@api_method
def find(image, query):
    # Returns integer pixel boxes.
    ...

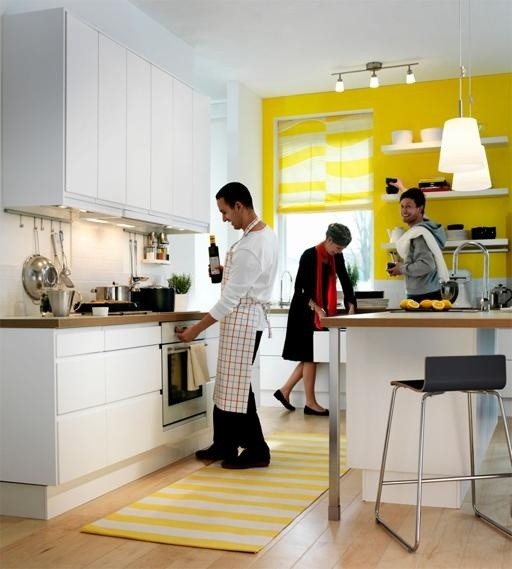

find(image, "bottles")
[495,283,512,308]
[144,231,170,260]
[208,235,222,283]
[131,287,140,303]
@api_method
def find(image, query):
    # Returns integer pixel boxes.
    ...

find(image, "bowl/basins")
[386,178,399,194]
[392,130,412,144]
[472,227,496,239]
[420,128,442,142]
[446,224,466,240]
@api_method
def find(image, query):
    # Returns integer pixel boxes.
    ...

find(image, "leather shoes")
[274,389,295,410]
[304,405,329,415]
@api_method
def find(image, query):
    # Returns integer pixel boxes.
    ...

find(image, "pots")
[22,227,58,305]
[91,281,134,302]
[140,280,176,313]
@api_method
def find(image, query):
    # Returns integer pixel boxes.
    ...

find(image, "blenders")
[440,269,472,308]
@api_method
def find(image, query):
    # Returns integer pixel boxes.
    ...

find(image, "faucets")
[453,241,490,312]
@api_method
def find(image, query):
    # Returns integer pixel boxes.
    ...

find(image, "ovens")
[161,319,208,433]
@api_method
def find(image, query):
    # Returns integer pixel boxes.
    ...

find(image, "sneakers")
[222,450,270,469]
[195,443,238,460]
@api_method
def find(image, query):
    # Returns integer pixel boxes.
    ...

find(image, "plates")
[354,291,389,310]
[418,177,451,192]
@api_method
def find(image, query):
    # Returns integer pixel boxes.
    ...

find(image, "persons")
[273,223,356,415]
[385,178,447,303]
[178,182,279,470]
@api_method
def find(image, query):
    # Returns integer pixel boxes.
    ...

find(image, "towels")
[185,343,209,391]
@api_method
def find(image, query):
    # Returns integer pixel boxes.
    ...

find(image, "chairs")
[372,355,511,553]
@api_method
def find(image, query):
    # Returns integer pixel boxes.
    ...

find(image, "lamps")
[452,1,491,193]
[437,1,482,174]
[330,61,420,92]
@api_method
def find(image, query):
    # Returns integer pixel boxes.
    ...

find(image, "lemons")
[399,299,406,309]
[431,299,445,311]
[406,299,420,309]
[420,299,433,309]
[440,299,452,310]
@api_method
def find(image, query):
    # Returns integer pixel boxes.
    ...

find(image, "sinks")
[391,308,480,313]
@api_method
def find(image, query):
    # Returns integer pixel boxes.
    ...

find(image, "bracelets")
[311,303,316,311]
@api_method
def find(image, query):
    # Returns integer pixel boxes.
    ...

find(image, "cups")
[39,288,83,318]
[92,306,109,316]
[14,301,27,316]
[478,123,488,138]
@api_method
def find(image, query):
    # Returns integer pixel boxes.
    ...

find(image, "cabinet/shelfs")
[207,321,260,425]
[53,323,164,487]
[0,6,126,222]
[98,45,172,233]
[172,73,211,233]
[380,136,509,254]
[260,311,346,409]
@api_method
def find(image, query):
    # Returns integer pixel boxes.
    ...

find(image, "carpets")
[78,430,350,555]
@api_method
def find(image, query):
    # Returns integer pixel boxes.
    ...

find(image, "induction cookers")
[72,302,139,314]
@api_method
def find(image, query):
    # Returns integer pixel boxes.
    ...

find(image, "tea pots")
[386,225,406,244]
